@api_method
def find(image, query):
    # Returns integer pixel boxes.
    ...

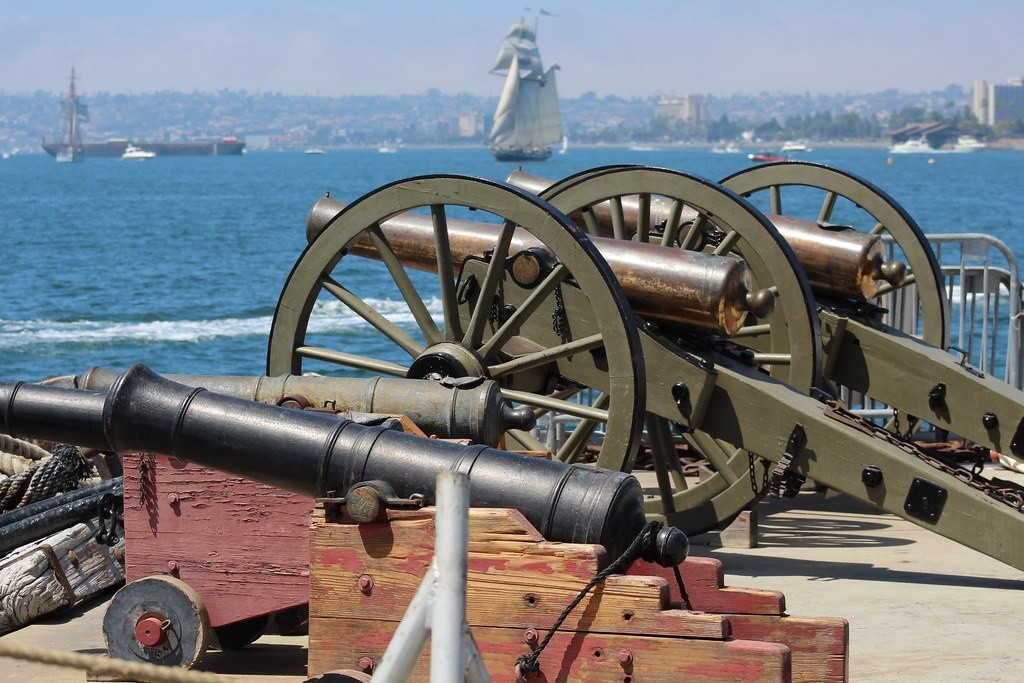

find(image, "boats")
[892,140,932,154]
[954,135,984,148]
[123,145,155,159]
[301,142,814,157]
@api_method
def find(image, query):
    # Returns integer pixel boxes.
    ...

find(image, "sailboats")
[42,65,244,157]
[489,10,565,161]
